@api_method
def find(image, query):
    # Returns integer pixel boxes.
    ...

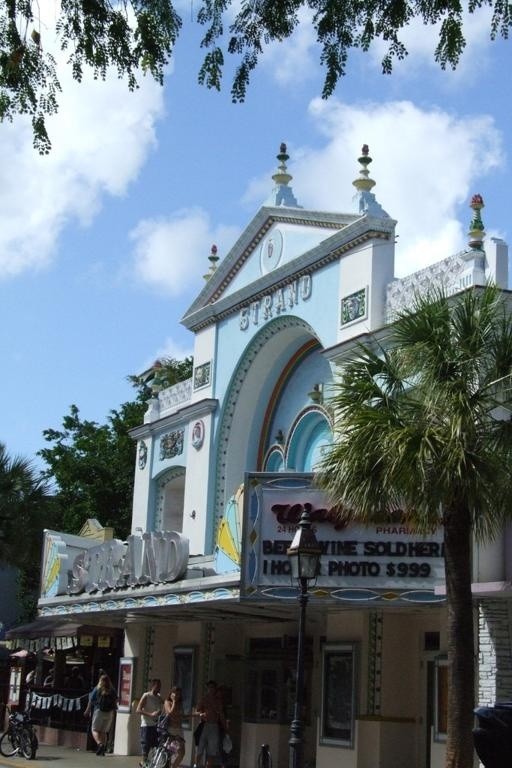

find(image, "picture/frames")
[338,284,368,330]
[169,644,197,731]
[318,639,359,750]
[193,358,211,393]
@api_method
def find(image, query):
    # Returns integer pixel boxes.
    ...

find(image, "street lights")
[286,507,321,768]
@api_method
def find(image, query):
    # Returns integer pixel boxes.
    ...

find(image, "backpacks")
[93,687,115,713]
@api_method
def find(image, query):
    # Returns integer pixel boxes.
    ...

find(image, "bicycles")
[0,703,39,760]
[143,726,186,768]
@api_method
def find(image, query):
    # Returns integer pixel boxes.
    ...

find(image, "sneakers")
[96,743,105,756]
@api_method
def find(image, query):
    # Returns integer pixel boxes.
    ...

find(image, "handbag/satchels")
[156,714,169,732]
[222,734,232,754]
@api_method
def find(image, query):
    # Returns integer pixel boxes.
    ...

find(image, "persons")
[134,676,166,768]
[161,686,199,767]
[196,678,232,767]
[23,661,118,756]
